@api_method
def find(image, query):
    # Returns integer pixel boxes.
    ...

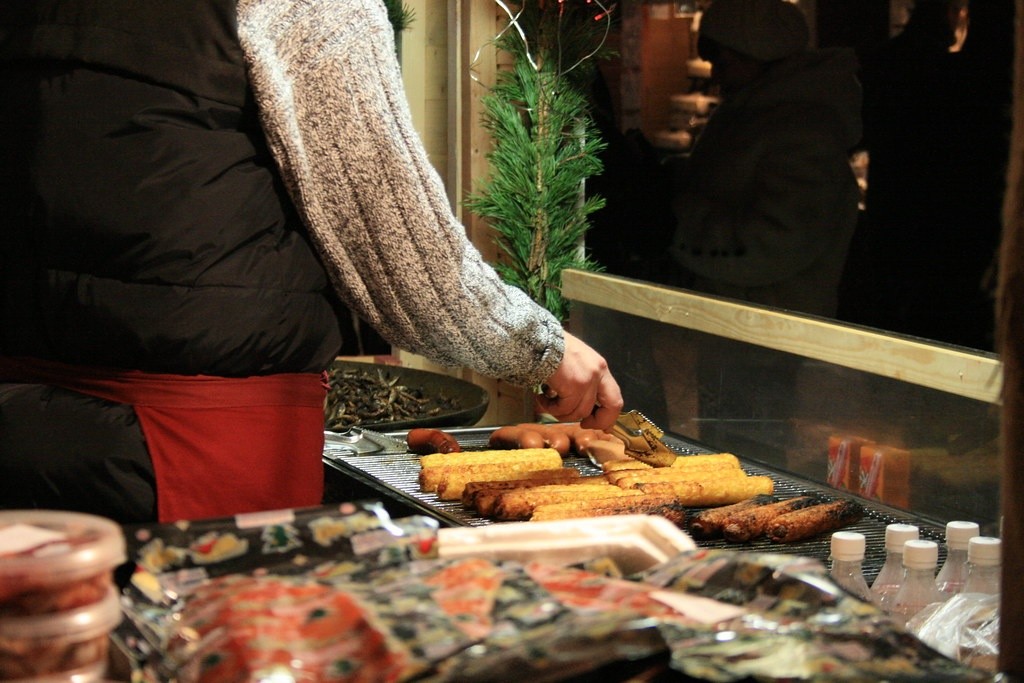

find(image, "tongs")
[542,384,675,468]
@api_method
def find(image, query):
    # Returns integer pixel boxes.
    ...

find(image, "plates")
[323,360,487,433]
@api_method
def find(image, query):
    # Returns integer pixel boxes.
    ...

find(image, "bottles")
[830,531,871,602]
[869,524,919,613]
[935,521,980,596]
[890,540,941,626]
[962,536,1002,618]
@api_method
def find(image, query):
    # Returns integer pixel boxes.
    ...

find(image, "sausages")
[404,418,627,456]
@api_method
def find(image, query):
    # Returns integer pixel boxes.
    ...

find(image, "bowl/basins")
[0,511,123,683]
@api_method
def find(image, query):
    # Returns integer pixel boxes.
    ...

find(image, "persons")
[676,1,865,464]
[0,0,624,527]
